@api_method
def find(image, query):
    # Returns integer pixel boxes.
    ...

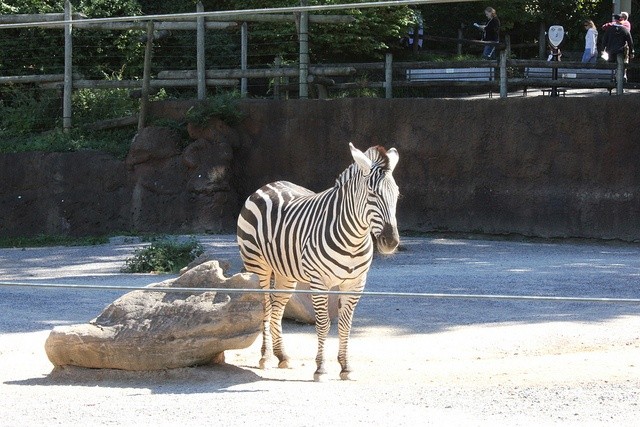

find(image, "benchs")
[406,67,494,98]
[522,67,627,96]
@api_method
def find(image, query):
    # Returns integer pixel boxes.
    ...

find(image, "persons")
[478,6,501,60]
[601,11,632,63]
[599,14,636,96]
[399,4,424,51]
[547,49,563,61]
[581,18,599,81]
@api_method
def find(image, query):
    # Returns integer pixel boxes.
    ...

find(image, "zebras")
[236,141,403,382]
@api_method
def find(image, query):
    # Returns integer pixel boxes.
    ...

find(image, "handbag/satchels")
[495,43,506,50]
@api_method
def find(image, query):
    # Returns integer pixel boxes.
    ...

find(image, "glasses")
[584,25,587,26]
